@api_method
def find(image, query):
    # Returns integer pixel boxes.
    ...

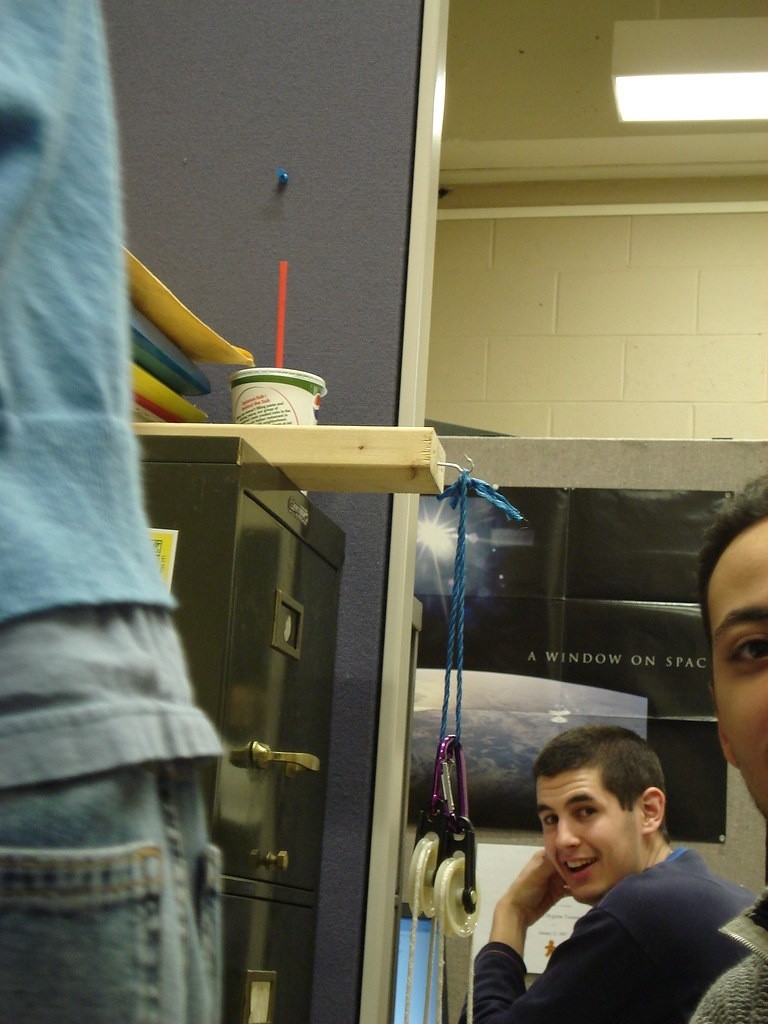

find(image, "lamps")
[611,0,768,123]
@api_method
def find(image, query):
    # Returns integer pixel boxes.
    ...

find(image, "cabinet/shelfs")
[134,435,346,1024]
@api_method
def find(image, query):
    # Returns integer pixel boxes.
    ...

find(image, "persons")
[687,469,768,1024]
[0,0,231,1024]
[456,725,756,1024]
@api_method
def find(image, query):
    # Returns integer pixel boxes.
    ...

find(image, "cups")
[229,368,328,425]
